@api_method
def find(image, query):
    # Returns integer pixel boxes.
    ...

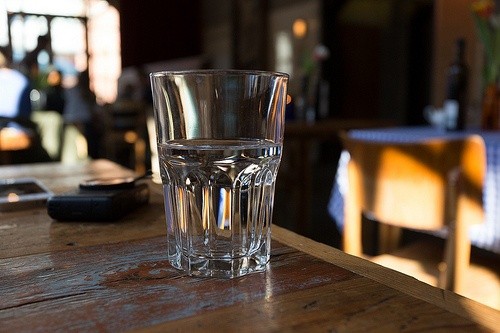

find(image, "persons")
[0,34,143,165]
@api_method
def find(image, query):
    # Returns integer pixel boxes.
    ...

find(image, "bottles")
[445,38,468,132]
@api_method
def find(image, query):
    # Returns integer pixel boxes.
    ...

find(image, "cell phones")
[0,178,53,212]
[47,183,149,222]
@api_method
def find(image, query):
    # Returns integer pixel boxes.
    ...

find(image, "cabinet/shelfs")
[110,1,434,241]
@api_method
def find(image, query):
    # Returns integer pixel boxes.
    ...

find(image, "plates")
[0,182,51,208]
[81,178,136,189]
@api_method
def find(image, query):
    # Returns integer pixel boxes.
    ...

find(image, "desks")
[0,164,500,333]
[342,129,500,294]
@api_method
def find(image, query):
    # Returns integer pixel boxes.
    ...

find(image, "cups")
[149,69,289,279]
